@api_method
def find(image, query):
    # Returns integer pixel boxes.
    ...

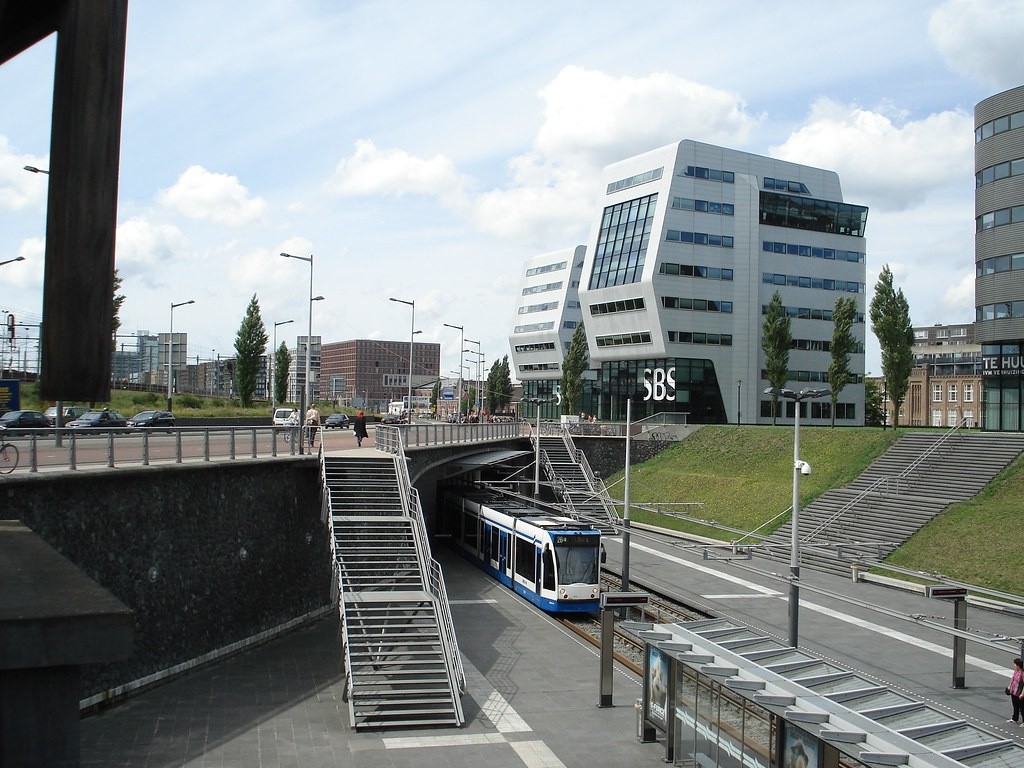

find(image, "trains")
[439,483,607,613]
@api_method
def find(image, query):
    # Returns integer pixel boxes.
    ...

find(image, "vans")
[44,406,91,427]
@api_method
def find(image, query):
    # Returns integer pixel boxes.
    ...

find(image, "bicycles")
[0,426,19,474]
[282,419,298,443]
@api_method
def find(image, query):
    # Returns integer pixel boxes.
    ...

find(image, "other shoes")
[358,442,361,447]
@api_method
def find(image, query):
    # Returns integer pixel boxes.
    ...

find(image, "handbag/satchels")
[312,421,318,428]
[353,431,357,436]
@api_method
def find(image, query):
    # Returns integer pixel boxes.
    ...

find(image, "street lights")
[389,298,423,432]
[449,338,489,415]
[735,380,745,427]
[168,300,196,412]
[761,386,832,648]
[272,320,295,418]
[280,252,325,438]
[519,397,558,508]
[442,324,464,423]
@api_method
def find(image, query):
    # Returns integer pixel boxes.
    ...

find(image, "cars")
[124,410,176,435]
[0,410,52,438]
[65,412,127,436]
[380,414,398,424]
[447,413,458,423]
[273,409,299,436]
[325,413,350,430]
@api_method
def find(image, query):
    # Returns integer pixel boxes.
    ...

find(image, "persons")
[1004,657,1024,727]
[305,403,321,448]
[287,408,300,427]
[353,410,368,447]
